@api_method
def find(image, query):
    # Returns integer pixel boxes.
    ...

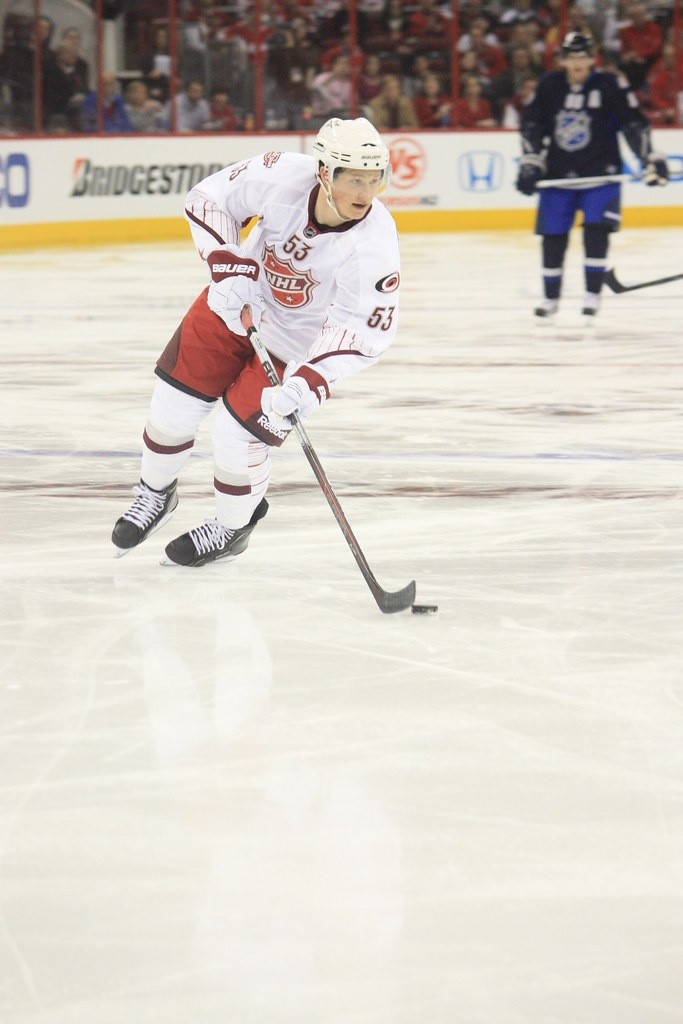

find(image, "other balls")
[411,604,439,615]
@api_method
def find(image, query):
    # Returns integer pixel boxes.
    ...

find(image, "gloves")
[207,247,264,336]
[515,163,542,196]
[261,359,332,431]
[644,157,669,186]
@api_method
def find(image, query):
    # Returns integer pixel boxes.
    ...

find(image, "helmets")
[311,116,390,195]
[558,28,597,59]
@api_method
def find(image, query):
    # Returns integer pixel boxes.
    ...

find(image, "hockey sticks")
[240,307,417,615]
[532,169,683,190]
[606,266,683,295]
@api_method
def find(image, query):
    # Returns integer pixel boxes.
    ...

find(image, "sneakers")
[107,478,181,557]
[159,500,270,573]
[534,294,560,317]
[580,292,603,315]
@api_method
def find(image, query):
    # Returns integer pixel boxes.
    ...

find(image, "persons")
[157,0,682,130]
[161,81,210,133]
[123,80,171,134]
[81,73,130,132]
[518,32,671,323]
[0,15,54,132]
[41,26,90,133]
[146,24,181,103]
[203,91,236,130]
[113,117,401,565]
